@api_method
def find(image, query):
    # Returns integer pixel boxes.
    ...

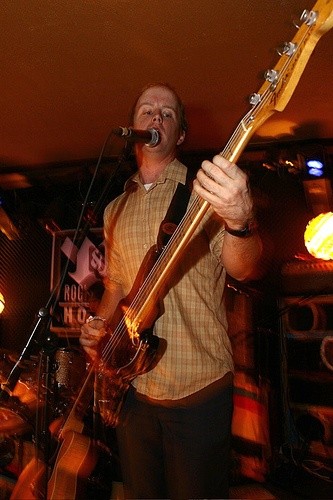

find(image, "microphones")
[113,125,159,147]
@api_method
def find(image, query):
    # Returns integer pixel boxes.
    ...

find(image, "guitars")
[9,365,93,500]
[89,0,333,429]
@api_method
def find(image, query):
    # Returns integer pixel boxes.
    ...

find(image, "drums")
[47,346,88,391]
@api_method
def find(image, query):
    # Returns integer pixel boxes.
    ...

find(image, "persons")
[78,83,276,500]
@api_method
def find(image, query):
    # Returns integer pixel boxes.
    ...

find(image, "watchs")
[225,218,258,238]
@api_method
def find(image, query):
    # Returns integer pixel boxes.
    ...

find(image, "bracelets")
[86,316,107,324]
